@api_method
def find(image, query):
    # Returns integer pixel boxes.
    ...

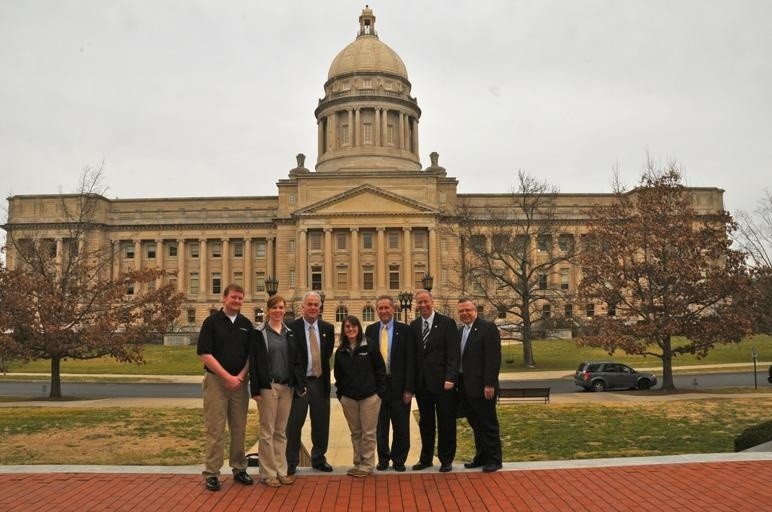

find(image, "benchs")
[496,388,550,403]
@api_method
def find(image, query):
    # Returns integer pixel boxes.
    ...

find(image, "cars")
[768,365,772,384]
[574,361,656,391]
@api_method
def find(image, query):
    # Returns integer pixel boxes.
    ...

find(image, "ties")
[380,325,388,365]
[422,321,430,349]
[309,326,321,378]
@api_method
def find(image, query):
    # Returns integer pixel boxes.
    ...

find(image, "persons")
[333,316,386,477]
[365,295,416,472]
[197,284,253,490]
[457,296,503,472]
[249,295,307,487]
[410,290,458,472]
[287,292,333,475]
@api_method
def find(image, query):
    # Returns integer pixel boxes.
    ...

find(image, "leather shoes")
[206,461,502,490]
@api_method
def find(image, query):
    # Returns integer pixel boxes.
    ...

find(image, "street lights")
[399,291,412,324]
